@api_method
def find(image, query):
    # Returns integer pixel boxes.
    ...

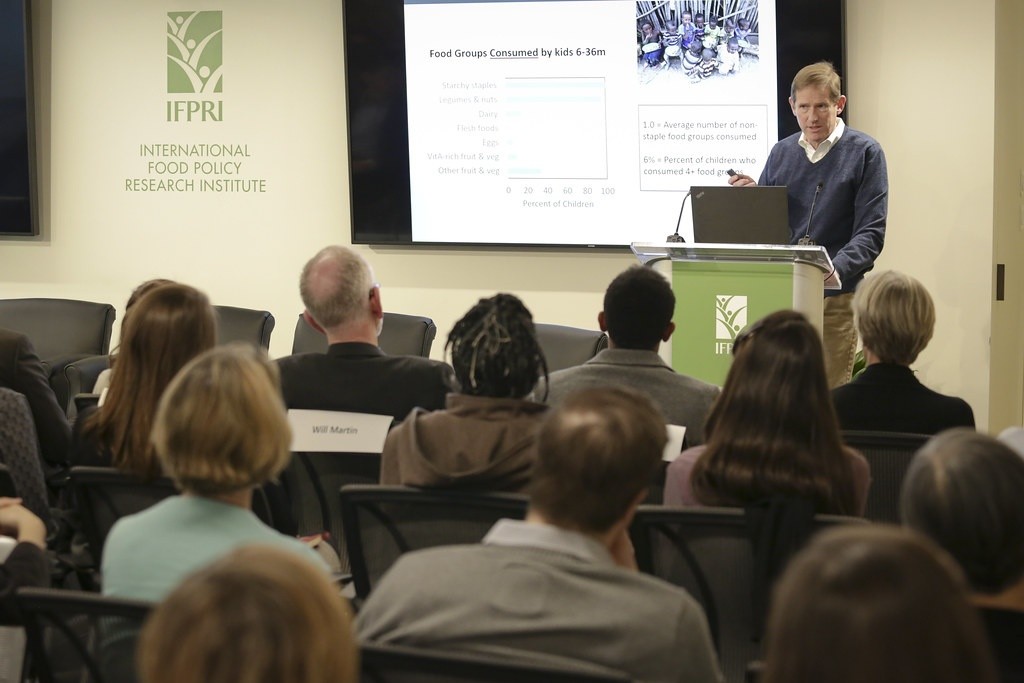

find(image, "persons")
[73,284,218,471]
[663,311,872,517]
[899,426,1024,683]
[832,270,976,434]
[0,329,70,636]
[357,385,725,683]
[639,11,751,79]
[728,64,889,391]
[274,247,452,428]
[100,342,343,602]
[380,293,554,492]
[533,266,718,449]
[135,536,357,682]
[763,523,1002,683]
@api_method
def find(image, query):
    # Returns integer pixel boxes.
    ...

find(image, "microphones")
[666,191,692,244]
[799,183,824,244]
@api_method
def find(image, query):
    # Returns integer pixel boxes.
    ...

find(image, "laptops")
[691,185,790,246]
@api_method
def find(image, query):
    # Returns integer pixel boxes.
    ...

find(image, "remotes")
[727,169,739,181]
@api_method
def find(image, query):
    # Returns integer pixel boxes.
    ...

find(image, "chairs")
[0,297,1024,683]
